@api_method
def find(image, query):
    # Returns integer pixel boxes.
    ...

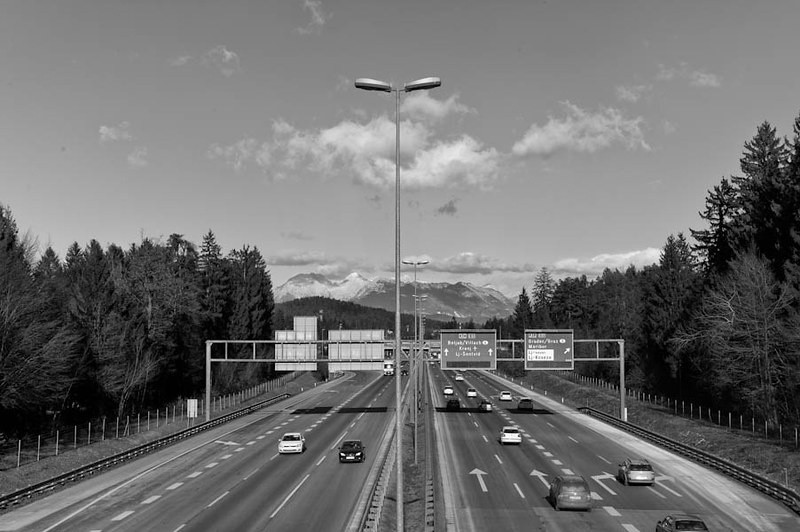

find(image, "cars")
[499,391,513,402]
[500,426,522,445]
[477,400,492,412]
[548,474,592,512]
[455,373,464,381]
[278,432,306,454]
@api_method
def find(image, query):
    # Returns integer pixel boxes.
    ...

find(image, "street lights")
[356,75,442,526]
[401,260,429,465]
[412,294,428,411]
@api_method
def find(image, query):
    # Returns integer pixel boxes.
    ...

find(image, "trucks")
[383,360,395,376]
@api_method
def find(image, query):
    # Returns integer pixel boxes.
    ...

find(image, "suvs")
[446,399,460,412]
[517,397,533,411]
[443,386,454,395]
[465,388,478,398]
[337,440,366,463]
[616,456,655,487]
[655,513,710,532]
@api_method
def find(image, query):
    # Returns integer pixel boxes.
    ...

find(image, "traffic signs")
[524,329,574,371]
[440,329,497,371]
